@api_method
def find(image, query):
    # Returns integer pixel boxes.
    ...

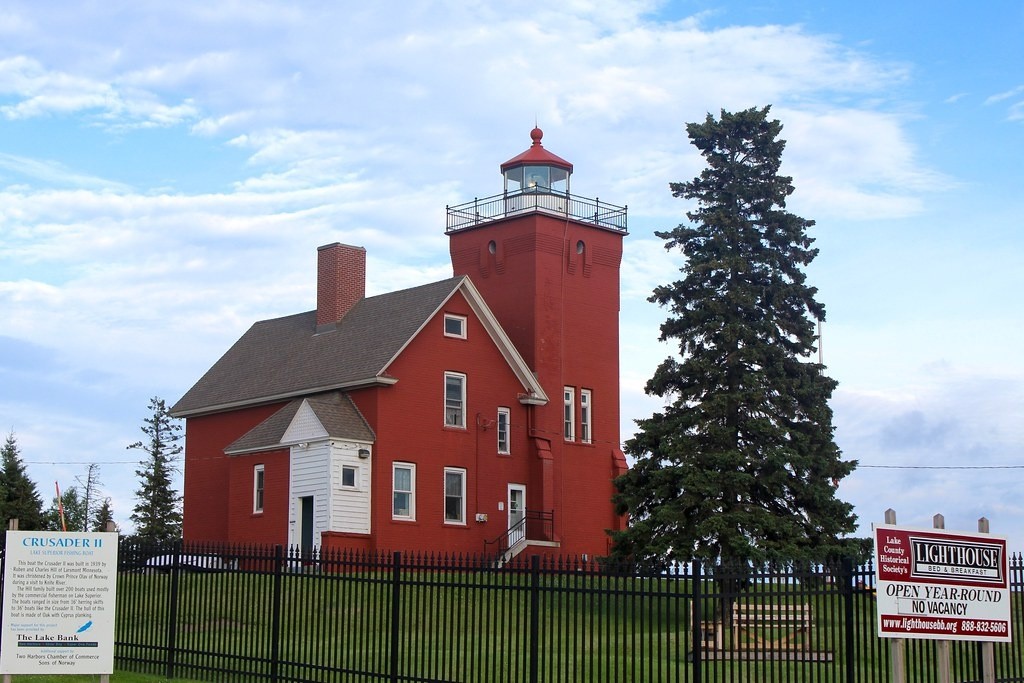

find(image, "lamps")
[359,449,371,458]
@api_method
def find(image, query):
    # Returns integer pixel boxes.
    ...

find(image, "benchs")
[733,601,815,651]
[690,600,723,651]
